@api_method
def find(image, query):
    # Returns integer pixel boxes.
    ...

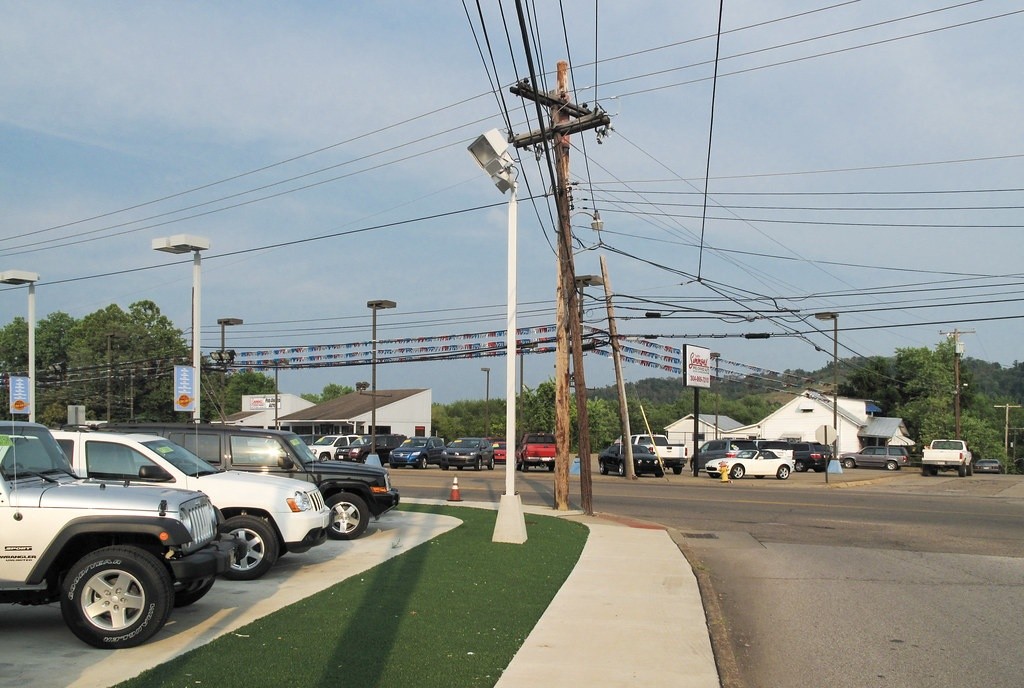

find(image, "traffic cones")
[444,471,465,502]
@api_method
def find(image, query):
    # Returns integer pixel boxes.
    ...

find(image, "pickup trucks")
[515,432,556,472]
[921,439,973,477]
[614,434,690,475]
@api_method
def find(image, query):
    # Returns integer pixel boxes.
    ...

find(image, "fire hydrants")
[717,461,732,484]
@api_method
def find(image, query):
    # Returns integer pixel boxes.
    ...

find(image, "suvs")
[839,446,911,470]
[790,441,835,472]
[46,422,335,581]
[690,439,758,471]
[0,419,249,649]
[752,438,796,466]
[292,434,506,472]
[98,417,400,541]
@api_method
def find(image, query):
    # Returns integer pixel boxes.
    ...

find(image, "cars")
[704,449,794,479]
[973,458,1006,475]
[598,443,666,477]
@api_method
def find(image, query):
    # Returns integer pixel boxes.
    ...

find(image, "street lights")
[575,274,605,344]
[518,343,539,440]
[151,234,212,424]
[710,352,721,440]
[272,359,290,431]
[480,366,491,439]
[466,127,530,543]
[814,312,839,459]
[0,268,40,424]
[105,332,126,424]
[366,299,398,454]
[215,318,244,423]
